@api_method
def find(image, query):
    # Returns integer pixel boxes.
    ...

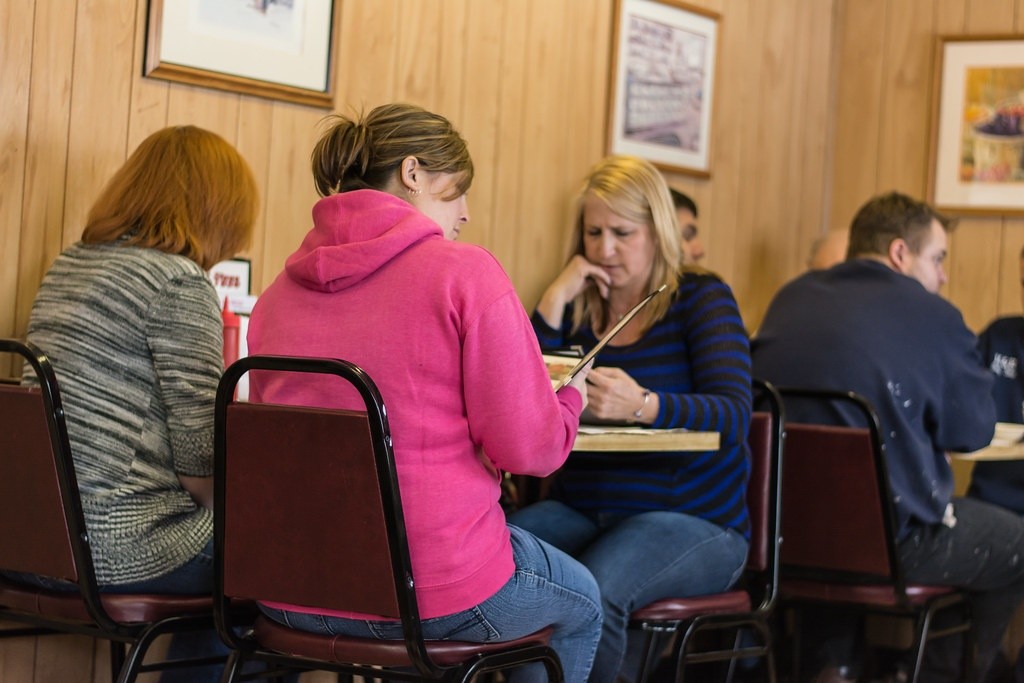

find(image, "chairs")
[624,377,786,683]
[0,336,302,683]
[775,387,978,683]
[211,354,565,683]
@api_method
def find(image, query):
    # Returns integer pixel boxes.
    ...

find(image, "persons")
[967,316,1024,514]
[751,192,1024,682]
[249,103,603,682]
[668,187,705,266]
[17,126,302,683]
[501,154,752,683]
[808,226,850,271]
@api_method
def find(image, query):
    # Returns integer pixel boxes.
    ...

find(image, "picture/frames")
[602,0,724,181]
[142,0,343,111]
[923,33,1024,221]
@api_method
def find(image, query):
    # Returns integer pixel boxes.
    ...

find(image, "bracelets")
[626,388,650,426]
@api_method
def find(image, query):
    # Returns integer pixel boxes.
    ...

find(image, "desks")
[944,443,1024,501]
[498,424,719,522]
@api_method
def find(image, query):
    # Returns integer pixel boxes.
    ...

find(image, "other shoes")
[811,659,860,683]
